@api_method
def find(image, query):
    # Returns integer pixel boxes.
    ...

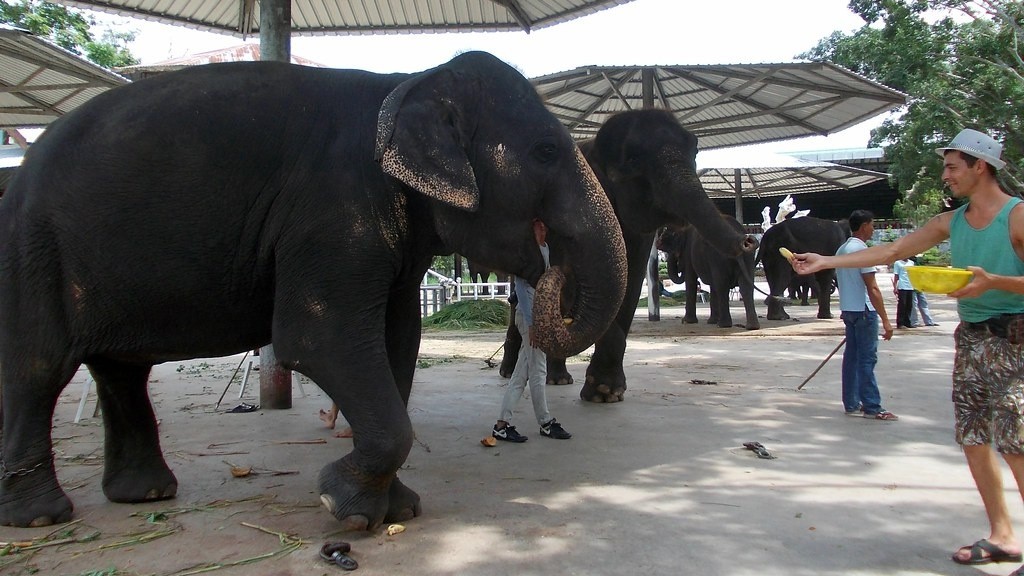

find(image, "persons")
[317,398,354,439]
[893,255,915,329]
[785,127,1024,576]
[492,218,572,442]
[908,256,939,328]
[831,209,899,420]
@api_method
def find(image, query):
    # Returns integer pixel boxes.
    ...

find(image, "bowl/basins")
[904,266,973,293]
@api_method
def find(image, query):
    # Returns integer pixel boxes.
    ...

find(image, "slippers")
[226,402,259,413]
[844,405,865,415]
[952,538,1023,565]
[863,411,898,420]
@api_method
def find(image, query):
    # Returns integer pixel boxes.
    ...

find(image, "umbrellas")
[520,58,911,321]
[693,154,894,229]
[1,23,133,132]
[43,1,637,412]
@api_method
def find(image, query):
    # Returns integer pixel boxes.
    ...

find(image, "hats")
[934,129,1007,171]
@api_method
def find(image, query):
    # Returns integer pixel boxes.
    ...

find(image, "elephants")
[0,50,854,533]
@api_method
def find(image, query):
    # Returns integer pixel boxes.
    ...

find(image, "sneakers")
[540,417,572,440]
[492,422,528,443]
